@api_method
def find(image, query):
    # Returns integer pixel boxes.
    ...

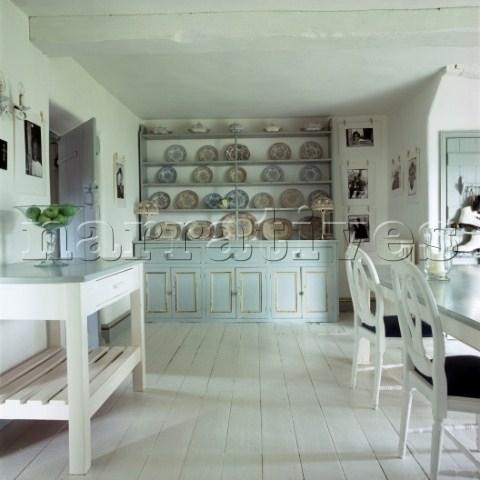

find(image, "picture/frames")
[345,212,373,244]
[390,162,402,192]
[343,125,377,150]
[25,120,43,178]
[405,154,419,195]
[113,152,126,200]
[342,165,375,206]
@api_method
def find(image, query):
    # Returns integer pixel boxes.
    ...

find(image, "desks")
[358,264,480,365]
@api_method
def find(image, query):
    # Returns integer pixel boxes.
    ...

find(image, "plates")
[150,143,258,240]
[259,141,335,240]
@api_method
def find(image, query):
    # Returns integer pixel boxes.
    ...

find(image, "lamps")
[135,200,159,240]
[12,82,31,120]
[312,196,335,240]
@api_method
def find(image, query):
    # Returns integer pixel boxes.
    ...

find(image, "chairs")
[344,245,433,410]
[390,258,480,480]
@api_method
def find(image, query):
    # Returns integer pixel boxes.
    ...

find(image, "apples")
[26,203,77,228]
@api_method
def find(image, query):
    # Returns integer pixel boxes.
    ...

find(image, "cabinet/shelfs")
[132,119,340,324]
[0,256,146,474]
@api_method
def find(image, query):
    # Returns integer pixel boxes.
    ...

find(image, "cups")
[423,228,452,281]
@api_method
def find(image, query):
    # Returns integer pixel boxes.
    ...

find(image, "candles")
[430,255,444,275]
